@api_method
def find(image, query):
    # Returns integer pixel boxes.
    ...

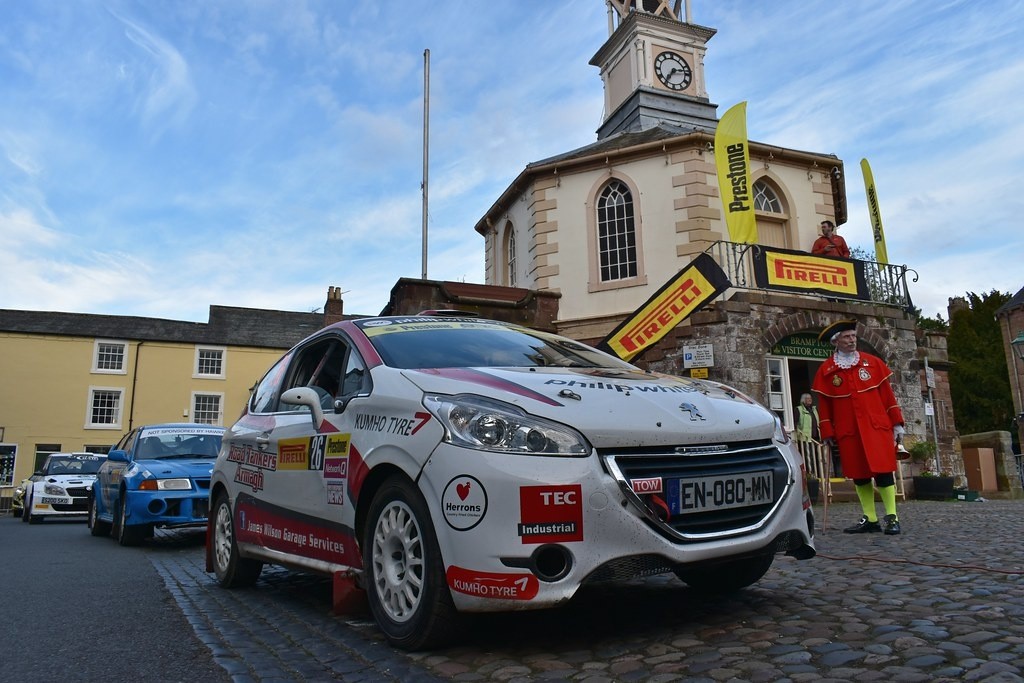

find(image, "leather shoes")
[884,514,900,534]
[843,515,882,533]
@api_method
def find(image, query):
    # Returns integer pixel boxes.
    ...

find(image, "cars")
[11,452,107,525]
[202,309,817,652]
[85,424,231,548]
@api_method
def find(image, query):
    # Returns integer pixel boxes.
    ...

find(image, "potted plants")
[909,440,955,500]
[806,472,819,506]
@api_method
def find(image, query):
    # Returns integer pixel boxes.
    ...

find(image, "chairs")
[146,438,162,455]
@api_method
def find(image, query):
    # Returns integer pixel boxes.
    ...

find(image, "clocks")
[654,51,692,92]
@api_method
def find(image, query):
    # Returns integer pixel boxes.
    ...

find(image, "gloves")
[824,440,834,446]
[894,425,905,440]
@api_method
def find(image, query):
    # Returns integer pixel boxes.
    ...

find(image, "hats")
[818,318,858,342]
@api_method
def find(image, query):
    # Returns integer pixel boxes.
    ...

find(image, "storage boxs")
[955,490,979,502]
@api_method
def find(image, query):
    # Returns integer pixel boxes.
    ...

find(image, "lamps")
[830,166,841,179]
[699,142,714,155]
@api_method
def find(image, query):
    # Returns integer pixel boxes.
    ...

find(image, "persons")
[811,221,850,304]
[810,318,905,535]
[793,392,822,477]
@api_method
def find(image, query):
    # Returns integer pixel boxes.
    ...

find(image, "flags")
[714,101,758,246]
[860,158,889,272]
